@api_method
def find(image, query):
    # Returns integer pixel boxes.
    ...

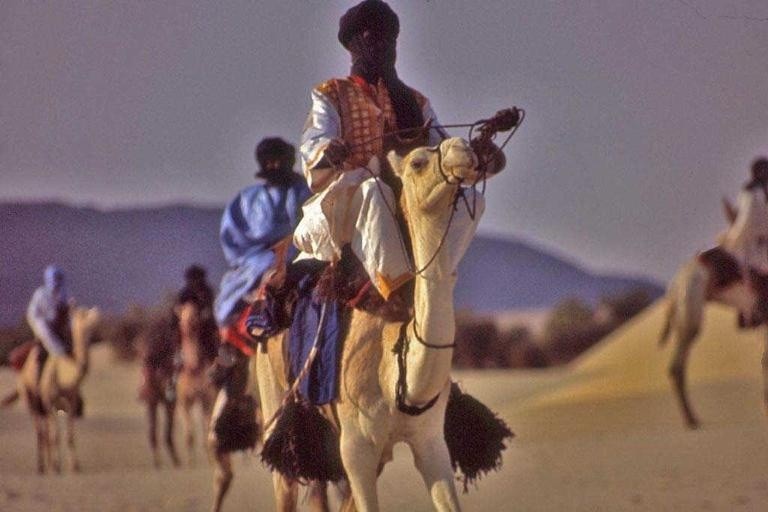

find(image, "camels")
[131,297,217,477]
[259,131,475,512]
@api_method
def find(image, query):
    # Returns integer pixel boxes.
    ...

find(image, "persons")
[0,264,83,417]
[715,157,768,280]
[205,135,312,386]
[140,264,215,402]
[293,0,520,315]
